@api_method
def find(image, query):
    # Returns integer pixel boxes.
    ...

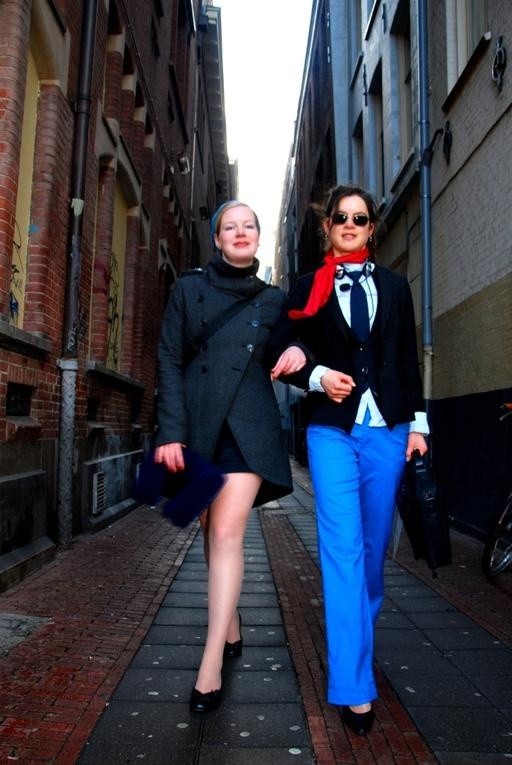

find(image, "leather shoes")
[224,610,243,658]
[343,703,373,735]
[190,687,222,712]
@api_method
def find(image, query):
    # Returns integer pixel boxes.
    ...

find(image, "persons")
[140,197,314,720]
[264,182,431,738]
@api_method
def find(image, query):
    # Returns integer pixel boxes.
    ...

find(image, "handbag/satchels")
[396,452,452,568]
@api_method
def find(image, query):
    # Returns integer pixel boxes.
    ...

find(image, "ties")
[345,269,370,395]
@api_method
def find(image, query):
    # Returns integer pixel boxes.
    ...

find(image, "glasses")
[330,213,368,226]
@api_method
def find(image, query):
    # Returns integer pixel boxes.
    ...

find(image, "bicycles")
[481,399,512,577]
